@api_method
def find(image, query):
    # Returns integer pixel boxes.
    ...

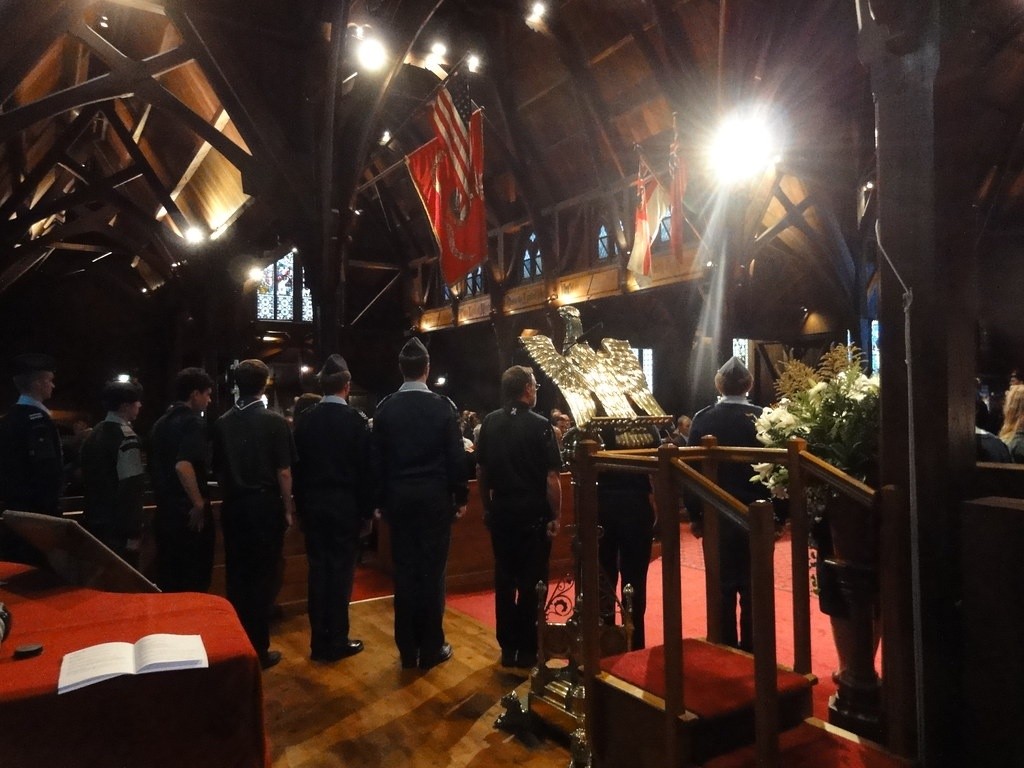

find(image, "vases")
[825,501,888,746]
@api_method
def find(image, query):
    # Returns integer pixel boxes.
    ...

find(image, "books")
[58,633,209,694]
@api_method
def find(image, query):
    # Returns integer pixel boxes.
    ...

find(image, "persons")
[688,357,787,654]
[673,415,692,521]
[975,366,1024,464]
[576,391,658,651]
[0,336,469,669]
[461,409,571,479]
[814,465,882,688]
[477,366,562,667]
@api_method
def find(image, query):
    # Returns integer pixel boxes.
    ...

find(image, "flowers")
[746,338,882,512]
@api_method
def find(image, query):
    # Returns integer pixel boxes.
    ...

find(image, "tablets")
[2,510,163,593]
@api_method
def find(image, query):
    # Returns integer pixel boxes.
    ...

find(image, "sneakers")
[261,651,281,669]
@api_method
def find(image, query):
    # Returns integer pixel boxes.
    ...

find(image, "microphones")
[562,323,605,355]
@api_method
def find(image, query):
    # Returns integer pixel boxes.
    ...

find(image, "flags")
[403,63,488,288]
[669,117,689,264]
[626,155,670,278]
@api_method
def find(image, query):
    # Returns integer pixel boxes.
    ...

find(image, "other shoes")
[502,648,538,668]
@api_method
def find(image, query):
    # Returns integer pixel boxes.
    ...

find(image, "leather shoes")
[402,643,452,669]
[311,640,364,662]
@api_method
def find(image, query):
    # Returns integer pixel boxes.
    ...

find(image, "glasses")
[535,384,542,391]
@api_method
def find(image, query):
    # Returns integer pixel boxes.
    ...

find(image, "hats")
[402,337,428,356]
[719,356,747,381]
[322,354,349,374]
